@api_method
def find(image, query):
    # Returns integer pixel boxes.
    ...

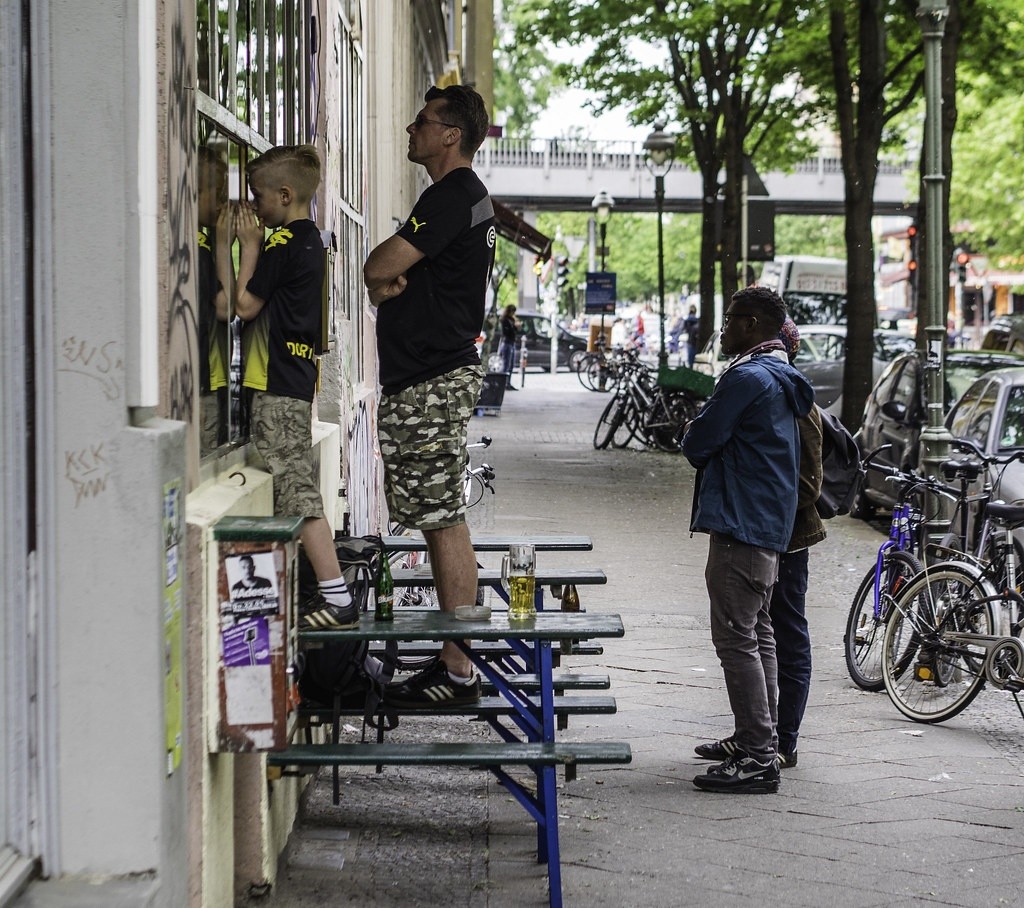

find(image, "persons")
[234,145,362,631]
[483,304,527,392]
[693,287,826,792]
[199,141,242,461]
[557,302,702,371]
[362,86,496,709]
[232,556,277,616]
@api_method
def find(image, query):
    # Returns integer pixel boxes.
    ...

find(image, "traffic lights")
[552,254,571,289]
[952,246,970,283]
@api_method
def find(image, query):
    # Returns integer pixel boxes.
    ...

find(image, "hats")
[777,312,803,359]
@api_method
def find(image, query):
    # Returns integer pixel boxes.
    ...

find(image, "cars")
[576,253,1024,588]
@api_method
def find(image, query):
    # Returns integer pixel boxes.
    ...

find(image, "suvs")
[476,308,591,373]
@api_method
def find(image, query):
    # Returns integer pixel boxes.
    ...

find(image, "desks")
[383,536,593,567]
[358,568,608,610]
[298,612,625,863]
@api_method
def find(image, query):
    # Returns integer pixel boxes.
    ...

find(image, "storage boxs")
[658,367,715,396]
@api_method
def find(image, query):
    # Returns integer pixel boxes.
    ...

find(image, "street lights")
[638,119,680,370]
[589,189,615,390]
[532,253,544,312]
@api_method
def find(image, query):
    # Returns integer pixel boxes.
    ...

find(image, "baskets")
[657,366,715,398]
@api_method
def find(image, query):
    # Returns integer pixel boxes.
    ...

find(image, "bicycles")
[881,499,1024,726]
[843,444,966,692]
[929,438,1024,692]
[353,433,497,670]
[592,347,700,454]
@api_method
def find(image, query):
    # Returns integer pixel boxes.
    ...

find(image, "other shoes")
[505,384,519,391]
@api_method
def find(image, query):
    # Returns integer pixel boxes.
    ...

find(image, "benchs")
[267,607,632,907]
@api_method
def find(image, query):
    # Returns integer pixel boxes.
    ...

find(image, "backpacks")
[298,536,399,730]
[816,402,866,518]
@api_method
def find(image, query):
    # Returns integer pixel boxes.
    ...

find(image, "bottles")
[374,552,394,622]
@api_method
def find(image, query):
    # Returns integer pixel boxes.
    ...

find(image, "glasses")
[722,312,761,329]
[415,114,464,132]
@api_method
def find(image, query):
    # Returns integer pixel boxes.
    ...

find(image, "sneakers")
[708,756,780,781]
[694,733,739,760]
[386,662,482,709]
[298,588,360,630]
[693,756,778,793]
[777,746,799,768]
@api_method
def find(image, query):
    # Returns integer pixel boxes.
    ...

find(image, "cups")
[500,544,538,620]
